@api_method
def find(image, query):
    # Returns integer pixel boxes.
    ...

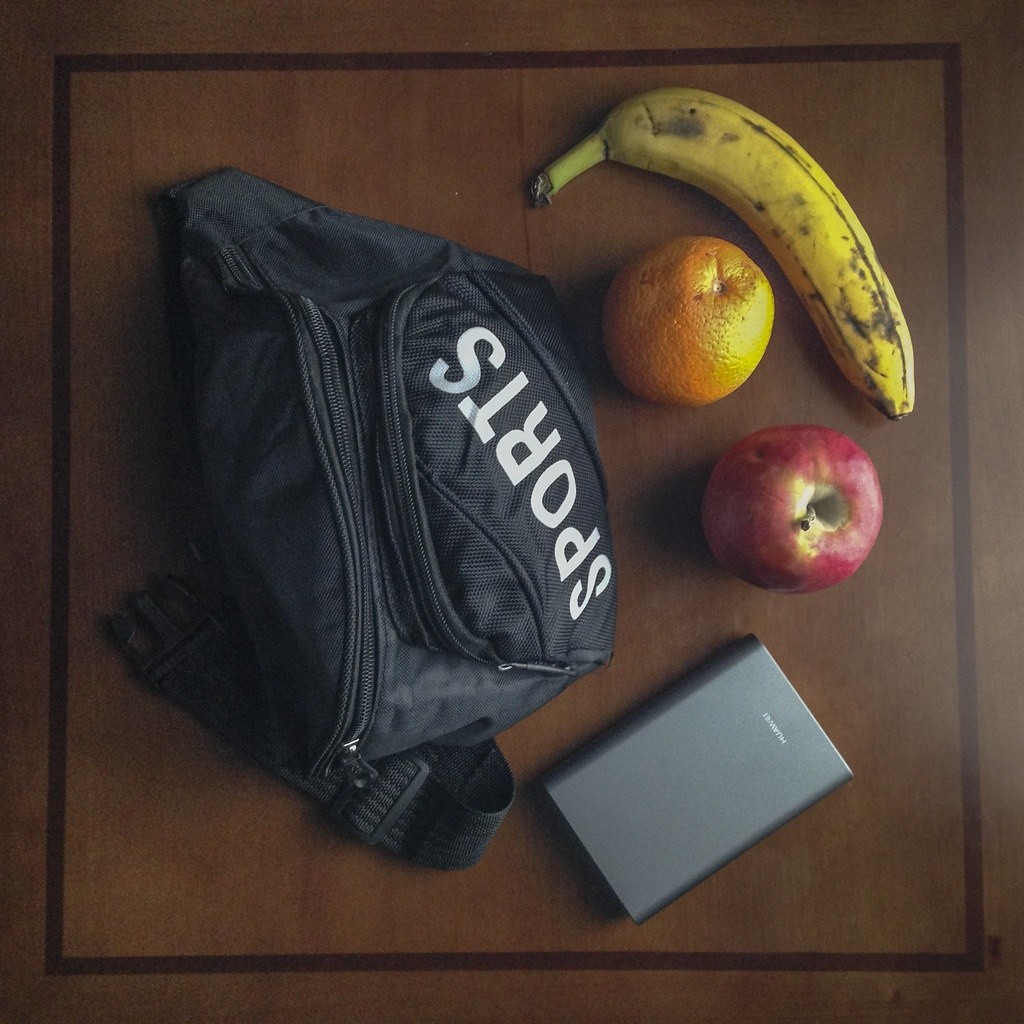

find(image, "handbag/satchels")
[112,168,615,870]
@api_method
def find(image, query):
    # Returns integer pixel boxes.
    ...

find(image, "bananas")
[529,88,917,422]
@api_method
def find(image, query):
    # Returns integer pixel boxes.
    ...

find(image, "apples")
[702,424,884,594]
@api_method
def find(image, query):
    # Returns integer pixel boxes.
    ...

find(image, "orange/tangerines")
[599,235,774,409]
